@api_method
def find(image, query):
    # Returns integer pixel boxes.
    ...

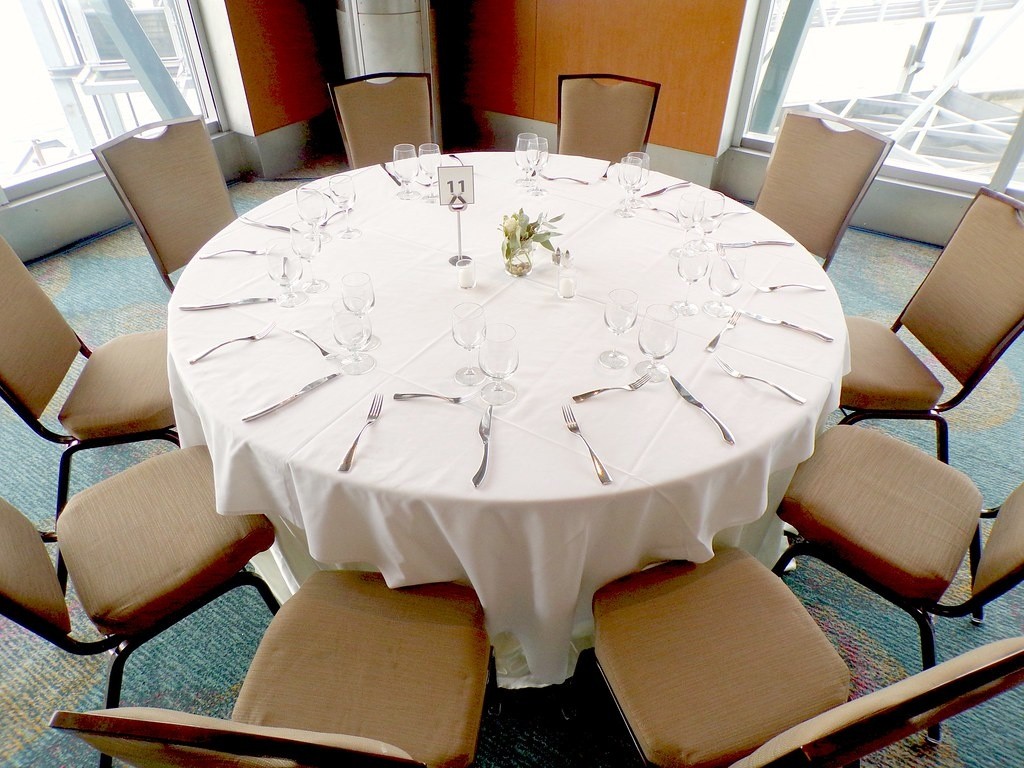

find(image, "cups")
[558,269,577,299]
[456,259,477,289]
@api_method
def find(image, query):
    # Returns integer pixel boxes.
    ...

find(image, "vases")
[503,249,533,278]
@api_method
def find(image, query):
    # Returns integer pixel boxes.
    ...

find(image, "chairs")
[0,72,489,768]
[557,73,1024,768]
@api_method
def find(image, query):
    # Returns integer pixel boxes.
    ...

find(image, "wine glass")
[670,243,745,319]
[598,288,679,383]
[451,302,519,406]
[392,143,442,204]
[669,190,726,260]
[514,132,549,197]
[264,175,381,375]
[613,151,650,219]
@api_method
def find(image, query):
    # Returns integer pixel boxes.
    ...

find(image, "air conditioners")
[336,0,444,155]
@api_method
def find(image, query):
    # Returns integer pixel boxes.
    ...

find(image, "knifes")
[242,373,341,423]
[670,376,736,444]
[239,216,299,233]
[641,181,692,198]
[471,404,493,488]
[380,161,401,186]
[720,241,794,248]
[742,310,834,342]
[178,298,276,311]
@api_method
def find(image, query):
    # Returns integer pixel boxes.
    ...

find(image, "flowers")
[495,206,565,258]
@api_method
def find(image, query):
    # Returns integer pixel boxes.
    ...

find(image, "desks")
[166,150,852,691]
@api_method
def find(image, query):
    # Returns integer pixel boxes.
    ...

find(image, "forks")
[705,310,742,353]
[713,355,807,405]
[188,322,277,365]
[293,329,339,360]
[599,161,615,181]
[561,405,613,485]
[539,173,589,185]
[338,394,384,471]
[393,393,478,405]
[571,373,652,403]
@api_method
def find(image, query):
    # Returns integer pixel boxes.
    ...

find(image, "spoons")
[749,279,827,292]
